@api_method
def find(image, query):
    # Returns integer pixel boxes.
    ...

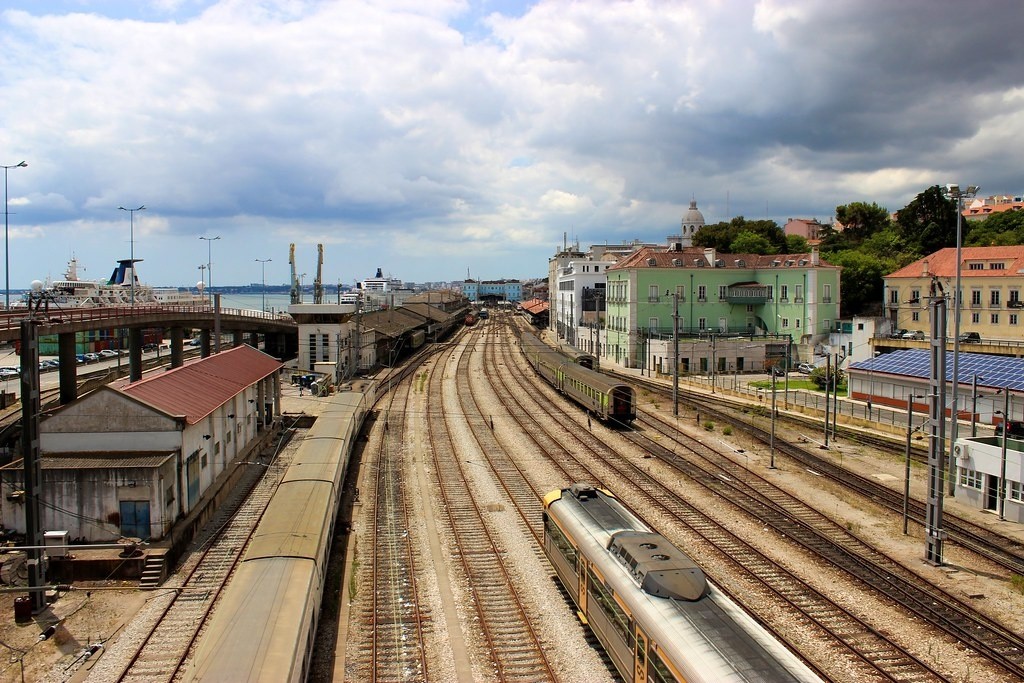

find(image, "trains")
[174,390,367,683]
[541,482,824,683]
[518,331,637,424]
[555,344,598,371]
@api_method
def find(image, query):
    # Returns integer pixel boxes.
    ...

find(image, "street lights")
[1,160,28,310]
[116,205,146,305]
[945,182,981,496]
[253,258,274,312]
[198,235,221,308]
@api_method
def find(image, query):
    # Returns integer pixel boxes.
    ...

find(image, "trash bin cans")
[13,596,33,623]
